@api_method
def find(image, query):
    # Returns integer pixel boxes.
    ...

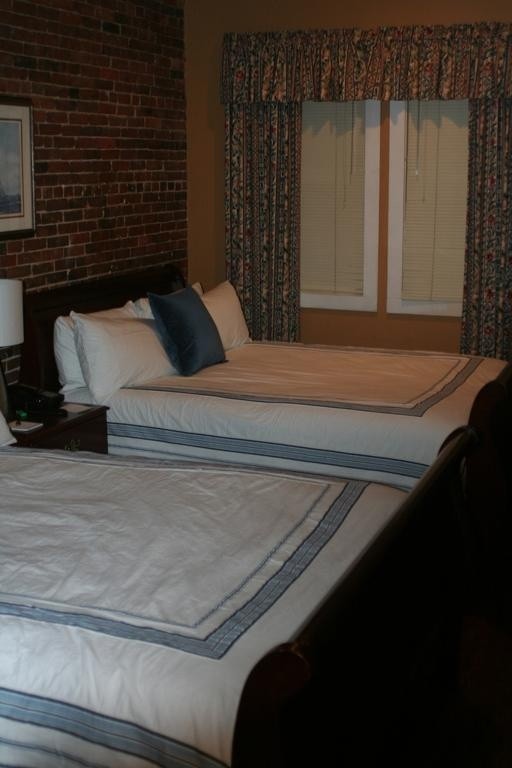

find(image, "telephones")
[6,384,64,410]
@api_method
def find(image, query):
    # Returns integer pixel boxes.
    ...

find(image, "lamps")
[0,278,44,433]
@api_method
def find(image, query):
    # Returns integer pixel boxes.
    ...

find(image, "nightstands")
[5,403,111,455]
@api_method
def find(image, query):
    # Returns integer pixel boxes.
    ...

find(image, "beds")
[2,426,510,763]
[17,263,511,497]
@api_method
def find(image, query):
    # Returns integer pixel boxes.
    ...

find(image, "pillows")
[144,284,230,378]
[52,300,141,394]
[137,279,254,350]
[69,308,180,408]
[133,283,205,320]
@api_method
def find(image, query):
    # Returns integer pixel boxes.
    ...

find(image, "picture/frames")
[0,93,38,244]
